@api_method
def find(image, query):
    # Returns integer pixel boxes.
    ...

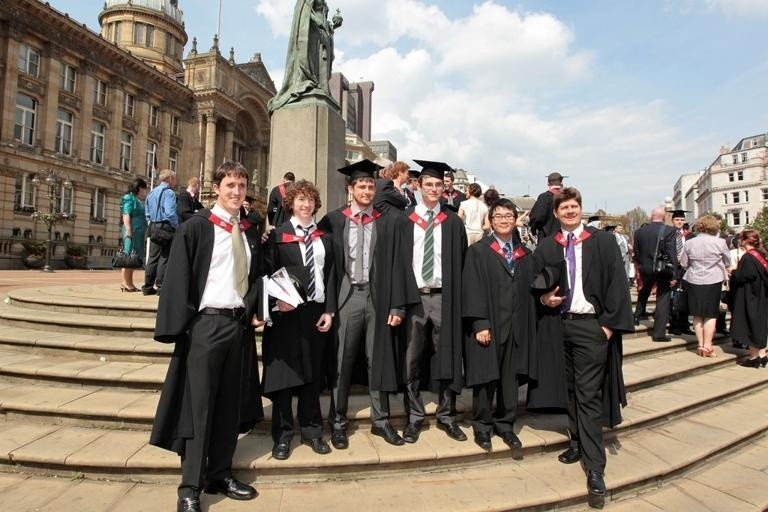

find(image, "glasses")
[492,214,515,221]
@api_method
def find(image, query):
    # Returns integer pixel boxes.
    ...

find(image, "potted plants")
[22,240,46,268]
[64,244,85,267]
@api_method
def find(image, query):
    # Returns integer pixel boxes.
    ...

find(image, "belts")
[201,307,238,317]
[418,287,442,295]
[566,313,598,320]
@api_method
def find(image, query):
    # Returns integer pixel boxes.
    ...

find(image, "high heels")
[697,346,717,357]
[120,284,141,291]
[740,356,768,368]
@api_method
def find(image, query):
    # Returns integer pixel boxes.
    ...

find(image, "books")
[261,267,306,312]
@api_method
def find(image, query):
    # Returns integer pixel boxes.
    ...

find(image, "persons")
[513,173,634,287]
[396,156,468,446]
[151,160,263,510]
[260,180,340,460]
[267,0,344,115]
[243,172,295,228]
[461,197,533,453]
[635,207,768,368]
[374,161,500,246]
[529,182,635,497]
[119,169,204,296]
[261,159,404,447]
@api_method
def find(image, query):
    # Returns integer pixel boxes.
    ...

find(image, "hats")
[666,210,692,218]
[603,226,617,232]
[245,196,257,204]
[588,217,599,224]
[409,160,456,180]
[337,159,385,182]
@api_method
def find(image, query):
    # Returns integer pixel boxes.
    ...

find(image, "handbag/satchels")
[112,249,143,268]
[719,281,730,312]
[150,228,173,246]
[672,292,688,311]
[653,253,673,280]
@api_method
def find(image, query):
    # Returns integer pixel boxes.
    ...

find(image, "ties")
[677,229,683,256]
[230,218,249,299]
[503,243,515,277]
[561,233,575,313]
[422,211,433,281]
[297,225,315,299]
[354,211,366,283]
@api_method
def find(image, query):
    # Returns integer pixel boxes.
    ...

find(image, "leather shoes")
[203,474,256,500]
[559,448,582,464]
[586,471,606,496]
[634,317,747,349]
[272,418,521,459]
[177,497,202,512]
